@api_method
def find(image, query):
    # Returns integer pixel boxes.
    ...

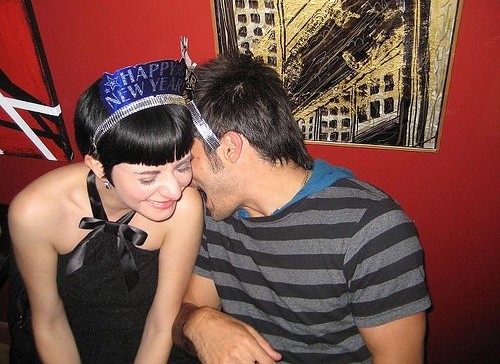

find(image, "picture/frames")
[210,0,465,153]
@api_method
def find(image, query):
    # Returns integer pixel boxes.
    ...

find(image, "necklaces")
[302,169,310,187]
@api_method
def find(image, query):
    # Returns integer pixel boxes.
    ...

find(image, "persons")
[171,53,432,364]
[8,36,204,364]
